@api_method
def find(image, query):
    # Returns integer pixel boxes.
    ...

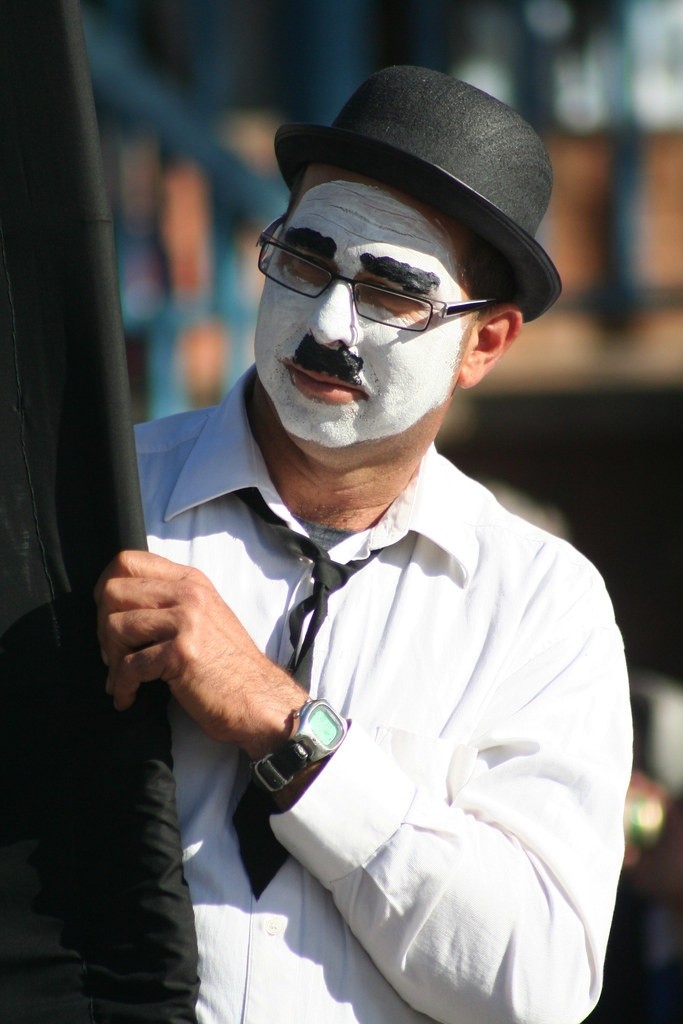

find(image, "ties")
[232,487,378,902]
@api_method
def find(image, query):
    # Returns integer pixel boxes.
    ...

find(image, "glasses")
[256,215,512,332]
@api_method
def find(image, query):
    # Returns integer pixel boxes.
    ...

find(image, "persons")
[95,62,632,1024]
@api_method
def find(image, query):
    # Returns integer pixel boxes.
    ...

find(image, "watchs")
[245,698,348,793]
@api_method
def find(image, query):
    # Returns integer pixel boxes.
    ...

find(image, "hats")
[273,66,563,323]
